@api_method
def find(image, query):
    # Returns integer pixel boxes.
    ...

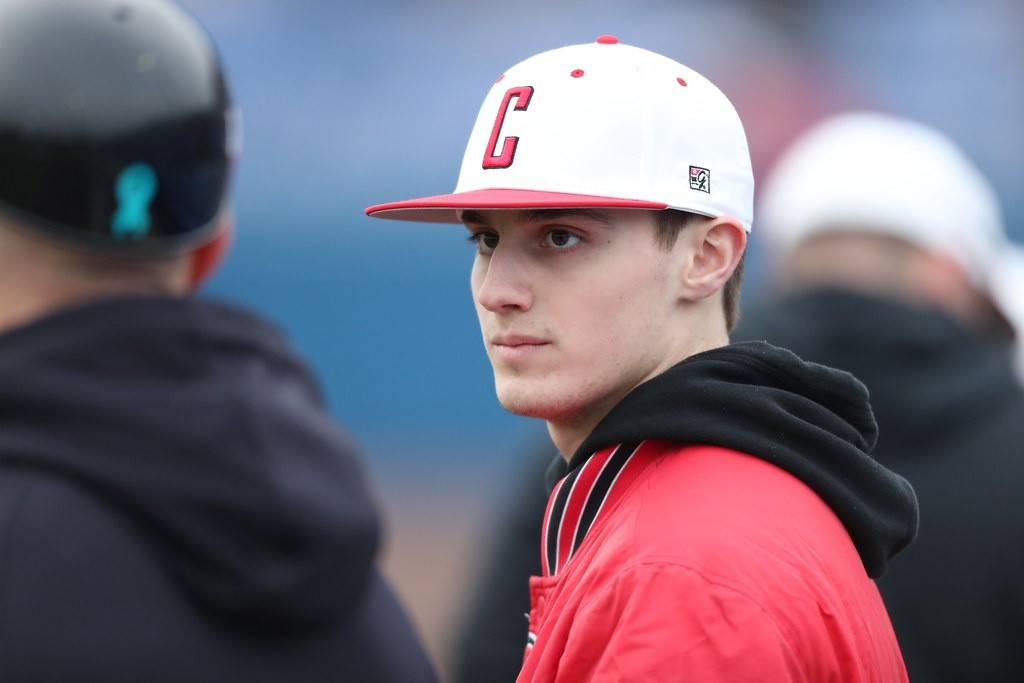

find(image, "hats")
[0,0,230,259]
[365,35,755,234]
[758,112,1024,337]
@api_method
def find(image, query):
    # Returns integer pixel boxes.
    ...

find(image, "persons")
[452,114,1024,683]
[366,35,919,683]
[0,0,441,683]
